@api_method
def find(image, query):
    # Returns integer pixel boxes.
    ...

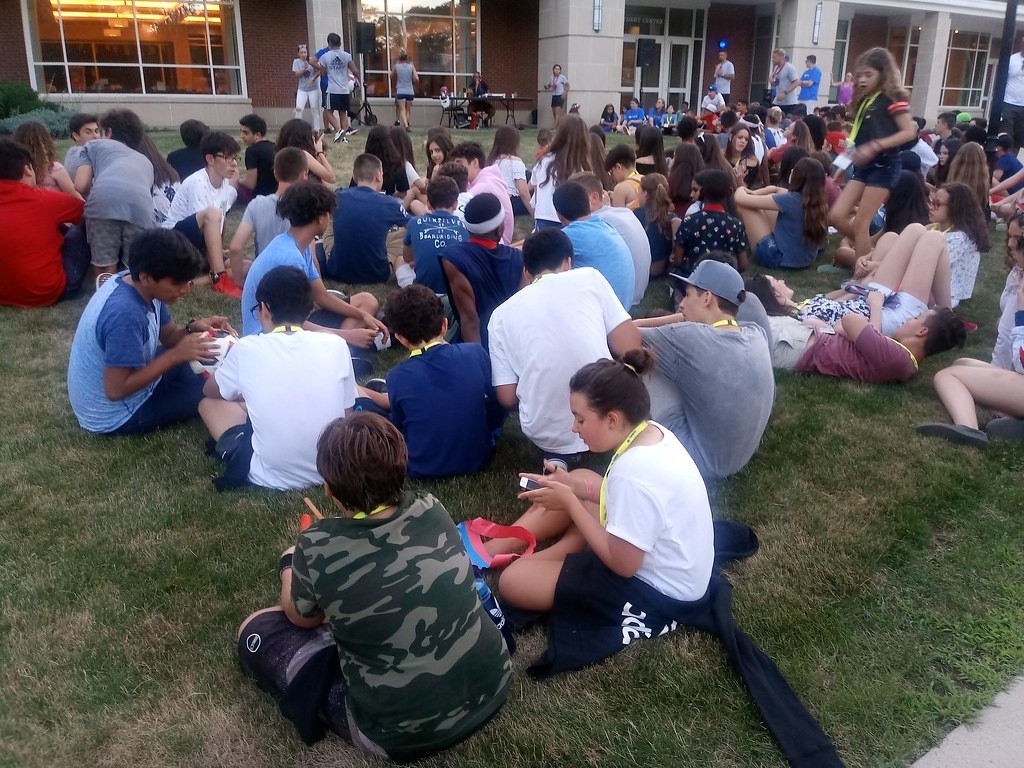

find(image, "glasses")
[608,163,618,177]
[212,153,242,163]
[298,45,306,48]
[932,199,951,211]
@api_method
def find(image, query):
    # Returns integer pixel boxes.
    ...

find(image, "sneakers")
[333,127,344,143]
[212,270,243,299]
[340,137,348,143]
[346,127,358,135]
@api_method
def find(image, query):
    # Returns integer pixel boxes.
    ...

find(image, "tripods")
[350,54,377,127]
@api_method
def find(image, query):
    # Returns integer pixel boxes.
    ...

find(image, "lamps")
[813,3,822,45]
[103,0,137,37]
[593,0,603,33]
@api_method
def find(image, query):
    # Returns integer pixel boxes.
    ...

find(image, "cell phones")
[520,477,543,493]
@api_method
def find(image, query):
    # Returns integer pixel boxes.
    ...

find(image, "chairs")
[440,98,492,127]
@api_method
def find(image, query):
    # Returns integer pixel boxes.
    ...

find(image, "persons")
[237,410,514,763]
[598,98,1023,278]
[766,48,862,105]
[292,33,360,143]
[0,105,1024,489]
[714,50,735,107]
[544,64,570,132]
[439,72,496,128]
[474,347,715,623]
[997,34,1024,148]
[390,46,419,132]
[701,83,726,119]
[826,45,918,271]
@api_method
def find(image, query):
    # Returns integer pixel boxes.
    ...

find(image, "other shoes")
[917,422,989,449]
[542,460,567,478]
[350,357,374,379]
[986,417,1024,439]
[366,377,387,392]
[96,273,114,291]
[394,121,400,127]
[959,318,977,331]
[406,126,411,132]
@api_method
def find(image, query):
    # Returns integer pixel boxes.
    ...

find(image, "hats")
[826,132,846,155]
[669,258,746,309]
[998,132,1015,149]
[709,85,718,92]
[704,105,717,115]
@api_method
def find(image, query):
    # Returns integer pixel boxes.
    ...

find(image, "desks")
[432,96,533,129]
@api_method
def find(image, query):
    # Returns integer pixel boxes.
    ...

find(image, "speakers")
[636,39,656,67]
[356,22,376,54]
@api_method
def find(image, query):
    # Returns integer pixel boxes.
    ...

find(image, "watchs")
[185,318,196,332]
[721,72,725,78]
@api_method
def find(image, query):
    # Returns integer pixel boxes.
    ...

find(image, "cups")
[600,119,605,124]
[512,94,514,98]
[451,92,453,97]
[463,92,467,98]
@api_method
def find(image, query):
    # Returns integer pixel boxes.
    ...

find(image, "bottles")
[475,578,516,655]
[458,88,462,98]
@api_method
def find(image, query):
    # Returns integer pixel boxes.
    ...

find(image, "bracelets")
[583,479,595,502]
[278,553,293,579]
